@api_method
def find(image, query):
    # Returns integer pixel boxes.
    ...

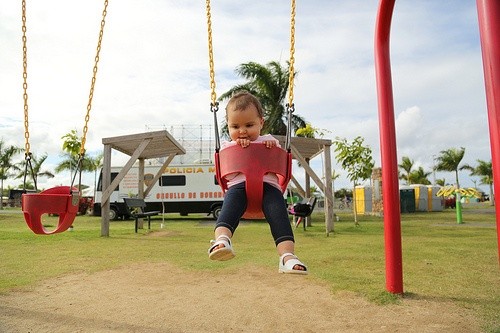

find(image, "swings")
[19,0,110,237]
[203,0,299,222]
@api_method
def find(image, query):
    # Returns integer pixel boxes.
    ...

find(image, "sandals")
[278,252,308,276]
[207,234,235,262]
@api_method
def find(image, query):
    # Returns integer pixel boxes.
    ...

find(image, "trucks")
[94,162,225,218]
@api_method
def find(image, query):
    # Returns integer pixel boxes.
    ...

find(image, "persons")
[208,89,309,275]
